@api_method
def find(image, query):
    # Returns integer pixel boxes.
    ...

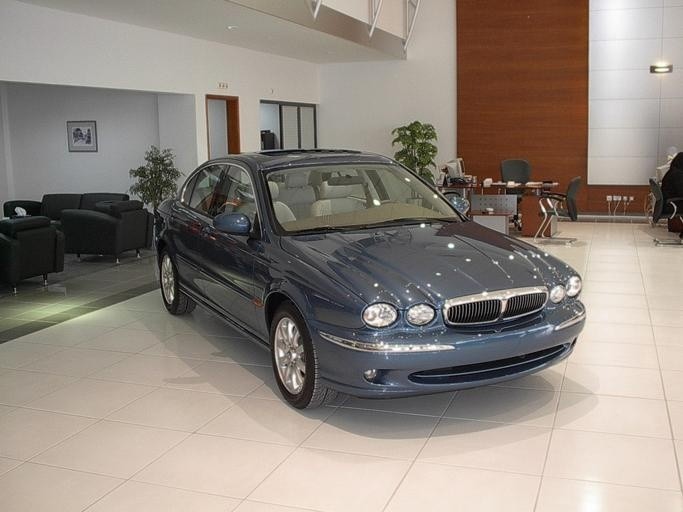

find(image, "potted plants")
[389,121,438,208]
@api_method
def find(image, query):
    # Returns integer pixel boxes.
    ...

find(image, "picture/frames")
[66,120,98,152]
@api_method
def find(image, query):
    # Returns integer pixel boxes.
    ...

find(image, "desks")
[434,182,559,237]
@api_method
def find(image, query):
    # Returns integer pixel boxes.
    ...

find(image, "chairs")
[235,172,363,225]
[0,215,64,295]
[500,159,580,244]
[60,200,154,264]
[648,177,682,245]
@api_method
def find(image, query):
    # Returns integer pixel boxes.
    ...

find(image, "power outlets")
[606,195,633,202]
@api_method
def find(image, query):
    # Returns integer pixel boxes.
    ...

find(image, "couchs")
[3,192,129,231]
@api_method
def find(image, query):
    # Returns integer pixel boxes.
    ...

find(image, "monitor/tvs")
[447,158,466,176]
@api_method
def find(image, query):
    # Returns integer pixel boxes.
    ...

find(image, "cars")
[148,143,590,414]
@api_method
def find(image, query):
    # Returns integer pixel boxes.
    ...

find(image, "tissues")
[11,206,31,219]
